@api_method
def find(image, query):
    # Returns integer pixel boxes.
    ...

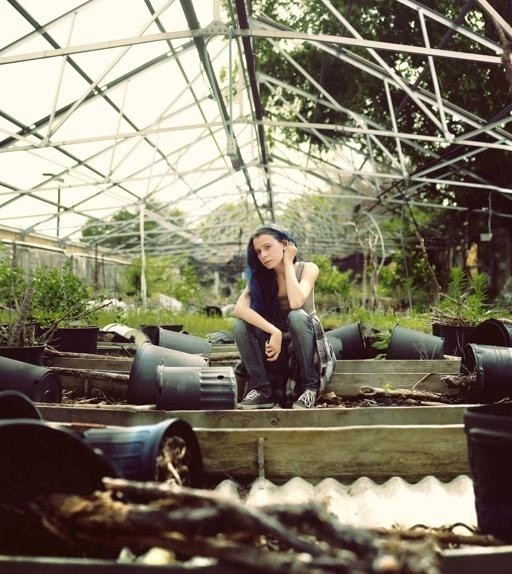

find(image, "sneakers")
[292,390,318,410]
[237,389,275,410]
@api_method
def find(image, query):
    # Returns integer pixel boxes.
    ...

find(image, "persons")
[230,223,338,410]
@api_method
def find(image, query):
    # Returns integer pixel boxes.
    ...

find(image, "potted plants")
[364,315,445,359]
[432,265,488,356]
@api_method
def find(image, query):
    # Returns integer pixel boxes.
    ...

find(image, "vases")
[325,323,363,360]
[1,419,204,521]
[460,317,512,545]
[59,325,100,355]
[103,322,245,408]
[1,342,64,420]
